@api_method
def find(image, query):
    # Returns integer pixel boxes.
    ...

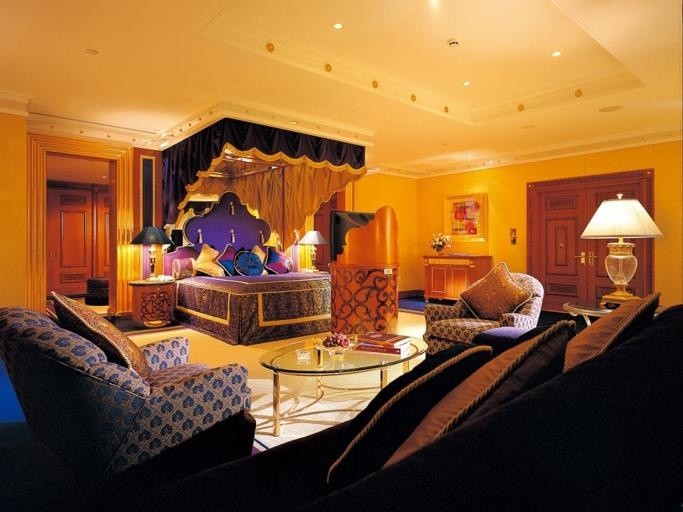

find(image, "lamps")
[577,191,665,306]
[296,231,328,276]
[127,225,176,280]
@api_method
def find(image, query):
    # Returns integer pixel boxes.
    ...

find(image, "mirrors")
[179,157,283,252]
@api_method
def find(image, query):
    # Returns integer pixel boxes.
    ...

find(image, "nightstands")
[129,280,178,328]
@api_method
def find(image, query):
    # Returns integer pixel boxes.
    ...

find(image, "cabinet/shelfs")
[419,255,495,303]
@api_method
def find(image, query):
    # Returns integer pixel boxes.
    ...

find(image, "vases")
[434,246,444,256]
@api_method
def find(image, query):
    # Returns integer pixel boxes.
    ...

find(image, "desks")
[563,303,660,326]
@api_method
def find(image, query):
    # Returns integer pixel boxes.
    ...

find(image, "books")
[360,342,410,354]
[359,332,411,346]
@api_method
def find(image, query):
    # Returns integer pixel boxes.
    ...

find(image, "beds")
[161,105,371,348]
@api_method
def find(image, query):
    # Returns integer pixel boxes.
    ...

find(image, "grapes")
[322,332,348,348]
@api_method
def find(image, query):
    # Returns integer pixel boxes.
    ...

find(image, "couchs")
[130,289,681,510]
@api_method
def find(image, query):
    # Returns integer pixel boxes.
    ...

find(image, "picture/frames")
[441,194,488,244]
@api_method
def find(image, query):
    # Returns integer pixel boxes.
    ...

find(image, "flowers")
[427,233,451,251]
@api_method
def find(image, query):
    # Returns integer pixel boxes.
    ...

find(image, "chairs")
[419,270,545,357]
[0,304,254,486]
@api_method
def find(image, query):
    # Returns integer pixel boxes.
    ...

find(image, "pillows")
[173,239,293,281]
[323,345,489,490]
[386,316,575,471]
[564,292,660,375]
[455,261,533,319]
[49,288,153,372]
